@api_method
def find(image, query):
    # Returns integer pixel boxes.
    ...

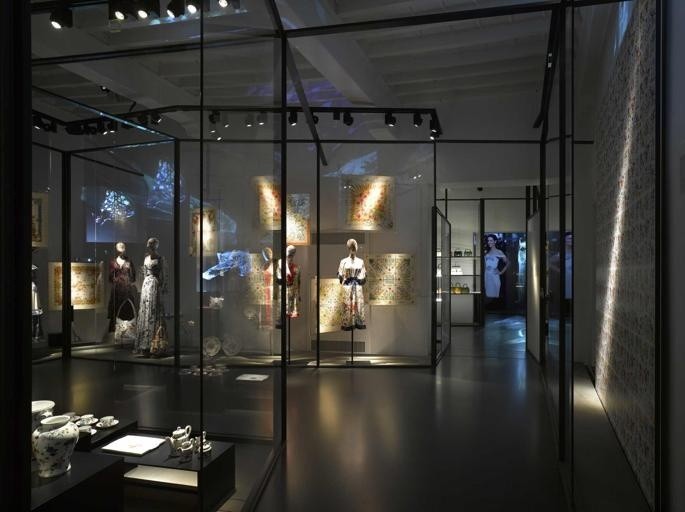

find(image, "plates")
[76,418,98,426]
[222,334,242,356]
[79,429,97,438]
[96,420,119,429]
[69,416,80,422]
[203,335,221,357]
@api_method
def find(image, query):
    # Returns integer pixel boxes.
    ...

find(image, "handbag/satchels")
[454,248,462,256]
[113,298,137,346]
[464,249,472,256]
[151,326,170,356]
[451,282,469,294]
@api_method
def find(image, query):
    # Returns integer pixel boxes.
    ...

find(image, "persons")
[107,242,139,332]
[135,237,166,358]
[240,247,272,331]
[338,238,367,331]
[277,244,304,319]
[484,234,511,298]
[32,265,44,338]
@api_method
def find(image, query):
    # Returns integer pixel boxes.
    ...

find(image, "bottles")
[32,415,79,478]
[32,400,55,459]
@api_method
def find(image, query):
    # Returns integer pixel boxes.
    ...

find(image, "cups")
[100,416,114,427]
[78,426,91,433]
[63,412,75,420]
[81,414,94,424]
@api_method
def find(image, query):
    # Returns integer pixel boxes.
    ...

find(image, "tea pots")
[165,425,212,463]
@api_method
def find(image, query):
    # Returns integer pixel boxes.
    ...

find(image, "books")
[102,434,165,456]
